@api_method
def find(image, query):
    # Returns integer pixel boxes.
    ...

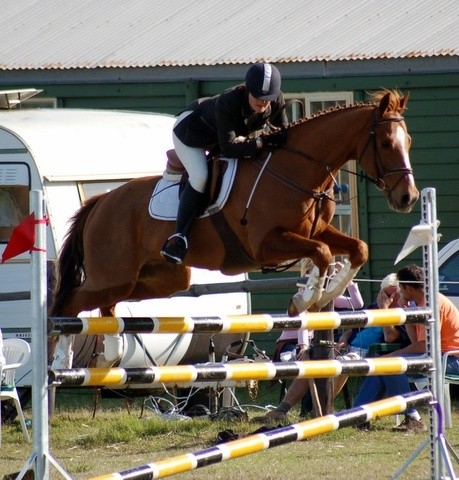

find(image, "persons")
[335,263,459,433]
[264,258,358,422]
[158,64,290,264]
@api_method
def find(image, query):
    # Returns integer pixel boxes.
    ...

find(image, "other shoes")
[266,411,285,421]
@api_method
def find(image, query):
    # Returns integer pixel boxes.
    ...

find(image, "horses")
[46,86,420,369]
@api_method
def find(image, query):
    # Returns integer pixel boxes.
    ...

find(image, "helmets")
[246,63,281,100]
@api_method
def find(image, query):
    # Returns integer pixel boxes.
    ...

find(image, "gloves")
[261,131,286,150]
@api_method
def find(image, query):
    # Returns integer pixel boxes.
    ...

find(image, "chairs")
[277,307,359,410]
[411,349,459,429]
[0,337,30,444]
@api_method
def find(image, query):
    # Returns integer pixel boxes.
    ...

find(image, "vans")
[436,239,459,311]
[0,87,251,426]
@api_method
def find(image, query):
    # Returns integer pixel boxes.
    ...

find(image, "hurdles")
[13,185,459,480]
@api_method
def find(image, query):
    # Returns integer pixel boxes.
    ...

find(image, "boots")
[162,181,207,263]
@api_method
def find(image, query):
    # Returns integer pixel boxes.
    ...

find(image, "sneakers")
[392,416,427,433]
[358,423,371,431]
